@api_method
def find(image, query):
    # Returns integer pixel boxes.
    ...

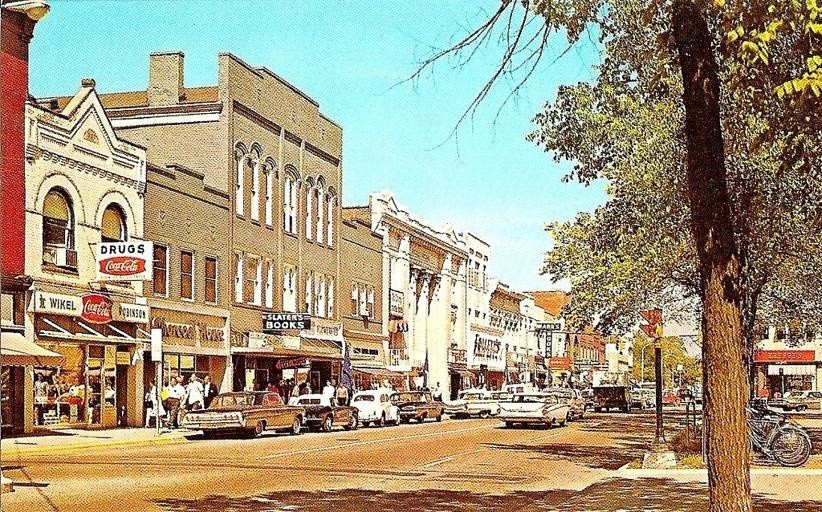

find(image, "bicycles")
[747,407,813,467]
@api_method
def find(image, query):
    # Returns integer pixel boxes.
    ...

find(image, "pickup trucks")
[179,391,306,438]
[389,390,446,424]
[290,393,359,433]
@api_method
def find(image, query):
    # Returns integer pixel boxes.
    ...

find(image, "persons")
[69,376,115,420]
[262,375,348,406]
[457,383,499,399]
[418,382,446,415]
[144,372,218,430]
[34,373,50,397]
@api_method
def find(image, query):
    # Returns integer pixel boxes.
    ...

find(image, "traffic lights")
[638,307,663,338]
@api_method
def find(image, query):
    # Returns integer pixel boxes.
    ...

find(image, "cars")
[350,389,401,427]
[781,390,822,411]
[445,390,503,420]
[503,382,703,416]
[501,392,571,431]
